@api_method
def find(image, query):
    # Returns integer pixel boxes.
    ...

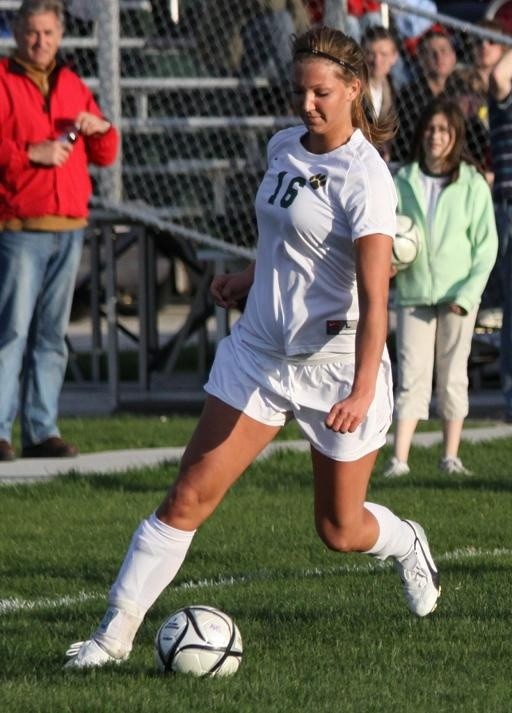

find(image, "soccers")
[157,606,244,677]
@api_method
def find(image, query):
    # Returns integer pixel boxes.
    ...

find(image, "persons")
[0,0,117,458]
[485,42,511,393]
[376,98,498,481]
[56,23,441,674]
[211,0,511,171]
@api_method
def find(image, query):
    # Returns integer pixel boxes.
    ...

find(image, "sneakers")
[399,516,442,613]
[0,438,14,459]
[61,637,122,667]
[440,456,467,474]
[384,457,409,479]
[22,437,79,458]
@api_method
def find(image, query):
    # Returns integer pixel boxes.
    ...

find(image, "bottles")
[52,122,81,145]
